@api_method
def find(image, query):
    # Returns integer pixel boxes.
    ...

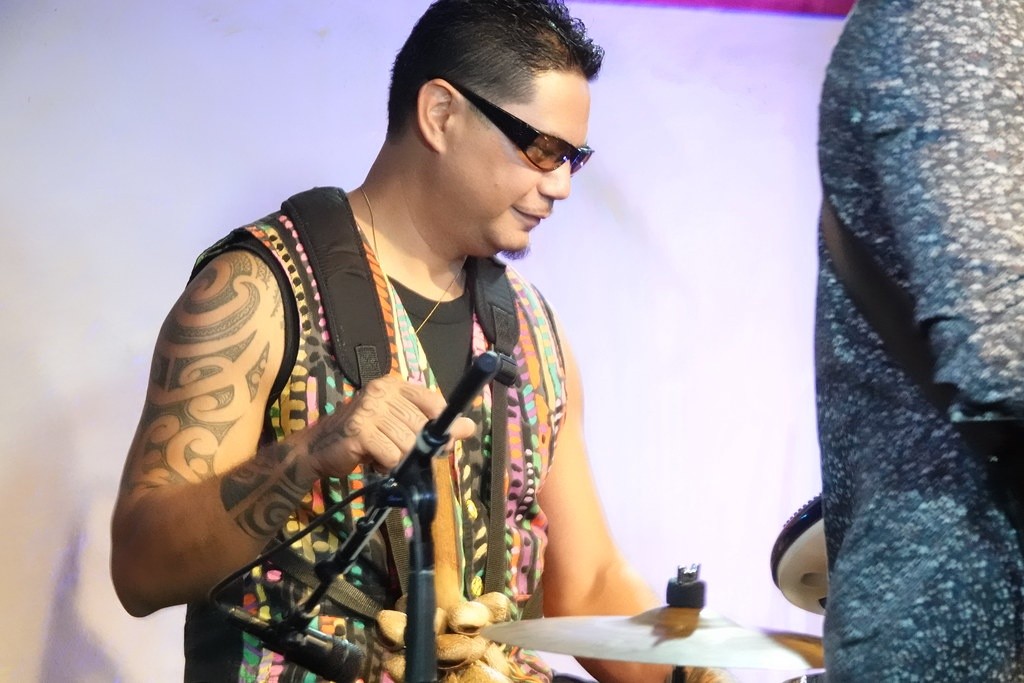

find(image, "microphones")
[213,603,364,683]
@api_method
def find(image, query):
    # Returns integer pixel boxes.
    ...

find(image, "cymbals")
[478,605,824,671]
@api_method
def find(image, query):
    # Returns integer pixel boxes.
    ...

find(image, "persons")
[813,0,1022,681]
[108,0,718,682]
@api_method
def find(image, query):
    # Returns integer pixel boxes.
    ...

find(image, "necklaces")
[360,184,464,339]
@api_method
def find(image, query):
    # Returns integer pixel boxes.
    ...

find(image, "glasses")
[414,76,596,176]
